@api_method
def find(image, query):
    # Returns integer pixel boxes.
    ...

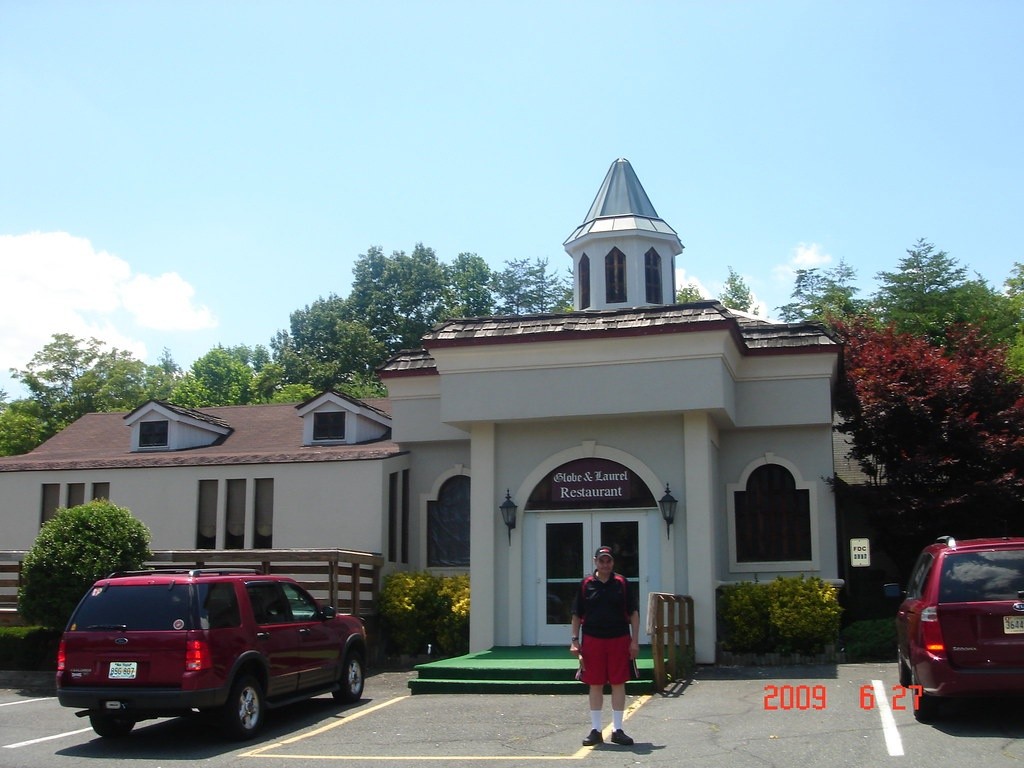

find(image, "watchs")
[572,637,579,641]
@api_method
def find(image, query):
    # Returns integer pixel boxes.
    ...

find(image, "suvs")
[884,534,1024,726]
[56,567,369,742]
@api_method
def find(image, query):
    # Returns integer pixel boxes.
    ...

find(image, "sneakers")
[611,729,633,745]
[583,729,603,745]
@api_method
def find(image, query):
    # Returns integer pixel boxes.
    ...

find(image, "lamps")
[657,482,678,540]
[500,489,518,547]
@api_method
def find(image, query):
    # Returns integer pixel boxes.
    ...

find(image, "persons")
[570,547,640,746]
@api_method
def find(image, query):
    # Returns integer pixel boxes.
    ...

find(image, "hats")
[595,546,615,560]
